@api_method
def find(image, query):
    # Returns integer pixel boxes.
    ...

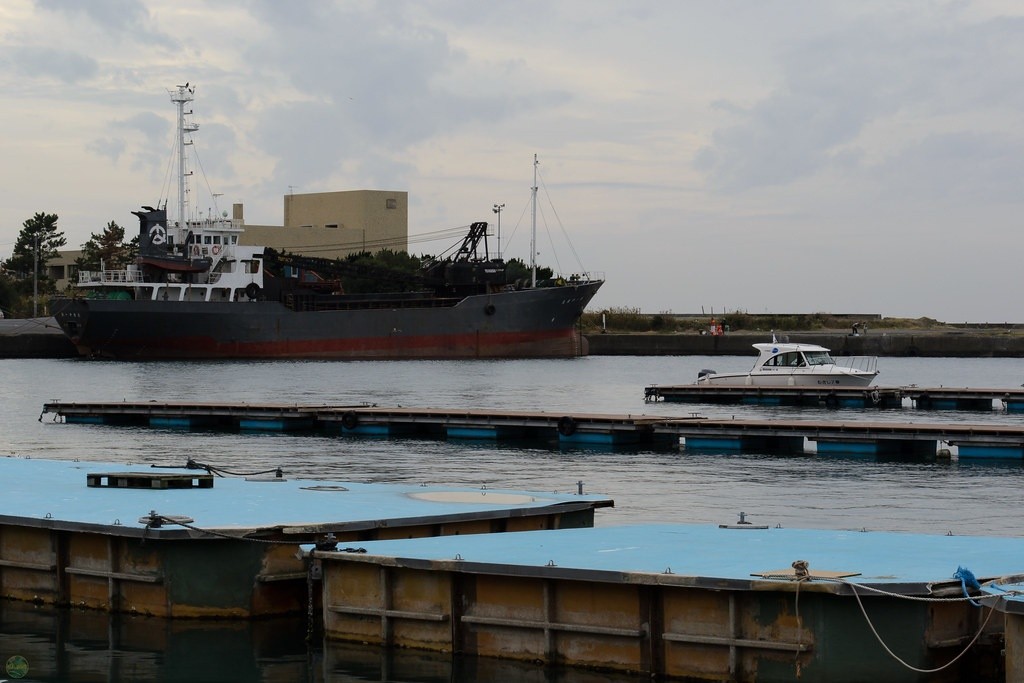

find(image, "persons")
[709,317,716,335]
[852,321,860,334]
[717,318,726,336]
[863,321,867,334]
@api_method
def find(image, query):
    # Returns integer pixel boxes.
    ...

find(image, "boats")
[46,83,610,360]
[695,333,880,388]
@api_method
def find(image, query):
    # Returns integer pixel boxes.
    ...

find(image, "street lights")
[493,203,505,259]
